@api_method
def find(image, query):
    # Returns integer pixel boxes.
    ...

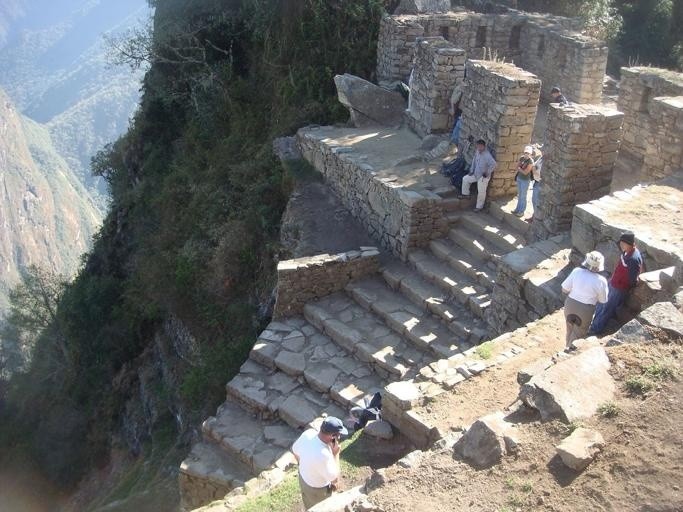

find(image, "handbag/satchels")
[451,168,469,187]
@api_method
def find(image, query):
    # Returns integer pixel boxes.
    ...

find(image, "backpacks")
[347,392,382,431]
[441,157,464,176]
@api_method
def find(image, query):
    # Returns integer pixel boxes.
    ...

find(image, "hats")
[620,230,635,245]
[537,143,544,151]
[524,146,533,154]
[582,251,604,272]
[323,416,348,435]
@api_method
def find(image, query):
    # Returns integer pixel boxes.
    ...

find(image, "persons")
[509,145,533,217]
[586,234,642,334]
[349,406,364,431]
[522,144,545,223]
[448,74,466,142]
[291,415,349,510]
[550,87,568,103]
[560,250,609,350]
[456,139,497,212]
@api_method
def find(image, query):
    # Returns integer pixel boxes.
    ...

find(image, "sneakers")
[511,209,524,217]
[457,194,471,199]
[473,208,481,212]
